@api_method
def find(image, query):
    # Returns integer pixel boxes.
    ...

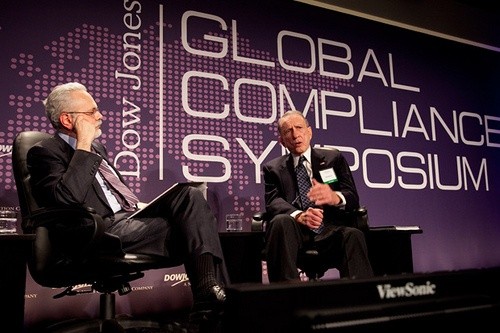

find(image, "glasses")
[63,108,99,115]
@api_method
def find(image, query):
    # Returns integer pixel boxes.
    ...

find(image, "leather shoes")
[195,283,226,303]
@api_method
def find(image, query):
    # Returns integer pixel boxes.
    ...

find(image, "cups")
[226,214,242,230]
[0,211,18,234]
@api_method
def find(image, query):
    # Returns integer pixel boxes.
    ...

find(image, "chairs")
[12,130,184,333]
[252,205,368,282]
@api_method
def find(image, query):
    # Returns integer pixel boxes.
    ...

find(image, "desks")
[218,231,263,286]
[368,225,424,275]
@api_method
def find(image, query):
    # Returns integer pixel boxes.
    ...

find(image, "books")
[368,225,422,231]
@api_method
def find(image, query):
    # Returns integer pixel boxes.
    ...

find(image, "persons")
[26,81,230,323]
[264,110,373,281]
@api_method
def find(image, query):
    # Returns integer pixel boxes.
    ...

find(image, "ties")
[91,147,139,211]
[294,156,324,233]
